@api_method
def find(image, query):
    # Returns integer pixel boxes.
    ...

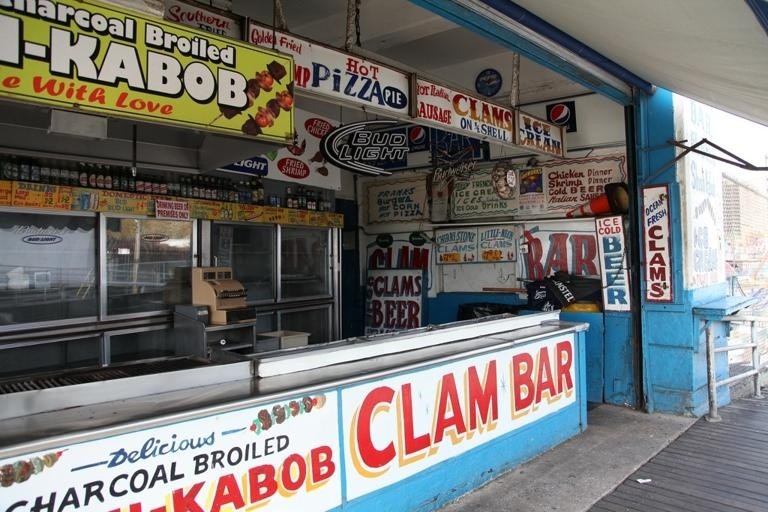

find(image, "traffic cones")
[566,181,629,218]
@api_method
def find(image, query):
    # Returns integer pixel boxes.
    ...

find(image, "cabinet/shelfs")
[0,205,344,381]
